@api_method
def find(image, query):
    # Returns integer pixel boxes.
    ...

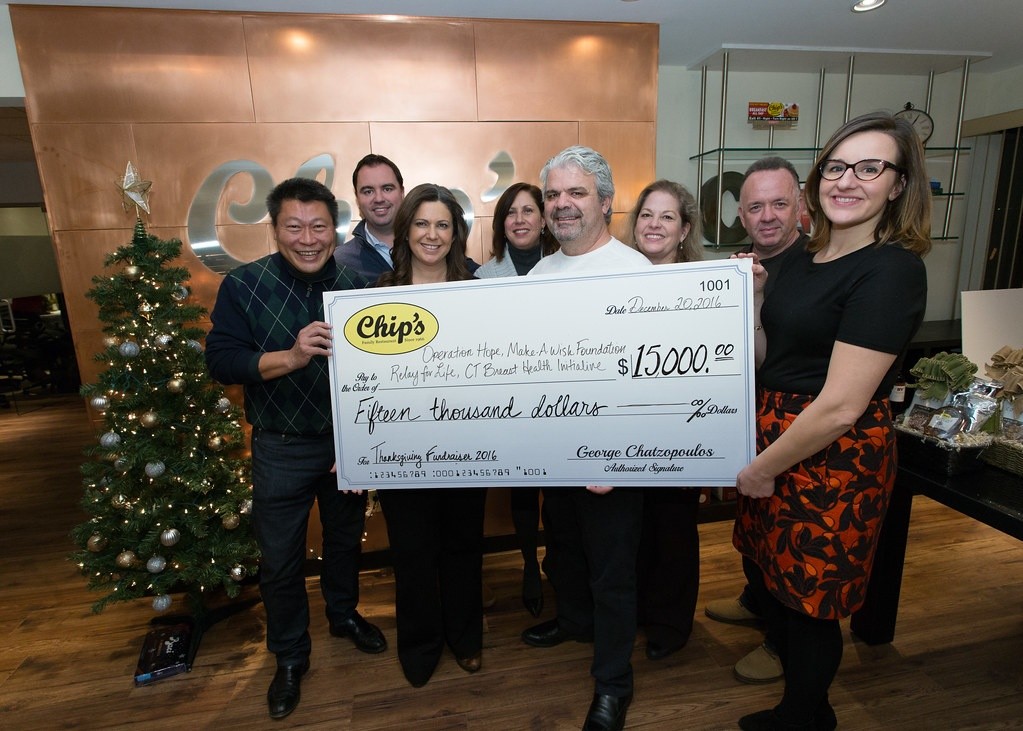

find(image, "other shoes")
[521,569,545,617]
[737,694,837,731]
[482,581,497,609]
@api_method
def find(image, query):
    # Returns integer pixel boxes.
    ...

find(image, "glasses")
[818,158,903,181]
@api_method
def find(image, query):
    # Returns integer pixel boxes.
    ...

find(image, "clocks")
[893,102,934,143]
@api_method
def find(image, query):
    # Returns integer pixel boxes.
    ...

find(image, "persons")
[623,181,701,661]
[728,111,934,731]
[204,177,388,718]
[473,182,560,621]
[522,146,654,731]
[378,184,484,689]
[332,155,404,289]
[703,155,812,686]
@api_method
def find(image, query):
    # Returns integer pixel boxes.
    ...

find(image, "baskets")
[979,430,1023,478]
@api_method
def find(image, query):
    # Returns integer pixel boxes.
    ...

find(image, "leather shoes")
[521,618,595,648]
[645,639,686,660]
[454,649,482,673]
[704,593,765,625]
[329,610,387,653]
[732,642,786,684]
[267,651,310,719]
[582,691,633,731]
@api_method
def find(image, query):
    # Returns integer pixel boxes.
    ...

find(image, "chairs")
[960,288,1023,381]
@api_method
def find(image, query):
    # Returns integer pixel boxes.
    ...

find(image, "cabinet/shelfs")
[892,320,961,421]
[688,44,993,250]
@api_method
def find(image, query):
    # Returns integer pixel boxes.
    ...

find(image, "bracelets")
[754,325,762,330]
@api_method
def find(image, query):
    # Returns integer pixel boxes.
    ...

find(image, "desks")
[850,462,1023,646]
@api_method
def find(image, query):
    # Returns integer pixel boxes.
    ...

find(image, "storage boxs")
[894,427,993,481]
